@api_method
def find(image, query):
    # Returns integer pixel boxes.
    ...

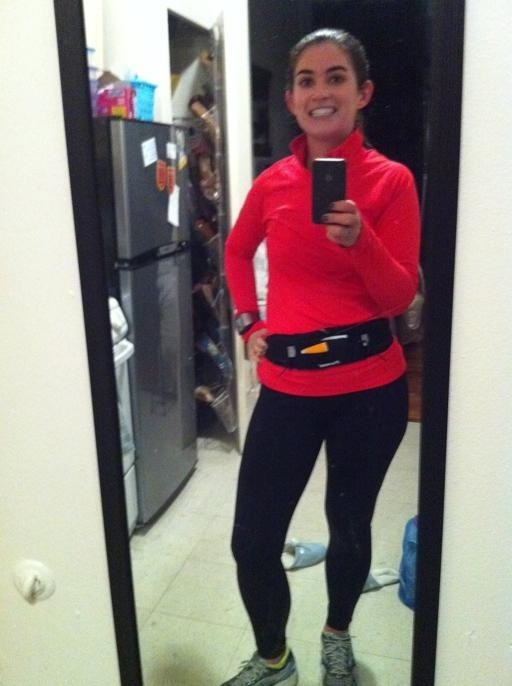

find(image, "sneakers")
[320,629,357,686]
[220,647,299,686]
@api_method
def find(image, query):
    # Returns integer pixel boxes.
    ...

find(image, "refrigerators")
[90,114,198,534]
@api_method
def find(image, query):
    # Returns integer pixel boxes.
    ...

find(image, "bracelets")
[235,310,260,336]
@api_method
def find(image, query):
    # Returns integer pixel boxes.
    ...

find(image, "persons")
[220,24,426,686]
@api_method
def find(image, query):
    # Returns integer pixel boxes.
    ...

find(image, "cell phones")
[312,158,346,224]
